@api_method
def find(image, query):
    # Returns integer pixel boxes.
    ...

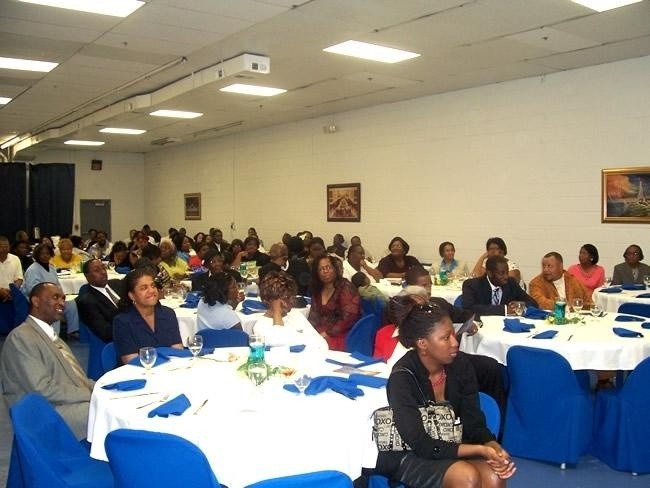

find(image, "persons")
[528,252,592,311]
[568,243,605,294]
[611,244,650,286]
[0,223,362,443]
[371,296,516,488]
[344,234,540,322]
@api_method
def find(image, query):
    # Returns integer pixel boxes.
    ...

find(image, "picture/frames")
[600,165,650,225]
[326,182,361,223]
[183,192,201,220]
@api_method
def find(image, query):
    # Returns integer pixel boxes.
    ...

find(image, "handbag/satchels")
[371,401,462,454]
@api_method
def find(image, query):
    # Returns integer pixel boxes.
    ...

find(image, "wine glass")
[362,251,480,294]
[51,246,262,299]
[138,331,315,410]
[512,274,650,325]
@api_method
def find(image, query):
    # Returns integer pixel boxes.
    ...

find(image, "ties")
[54,338,88,386]
[106,288,119,307]
[493,288,500,305]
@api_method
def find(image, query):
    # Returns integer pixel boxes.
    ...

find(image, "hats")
[202,248,219,264]
